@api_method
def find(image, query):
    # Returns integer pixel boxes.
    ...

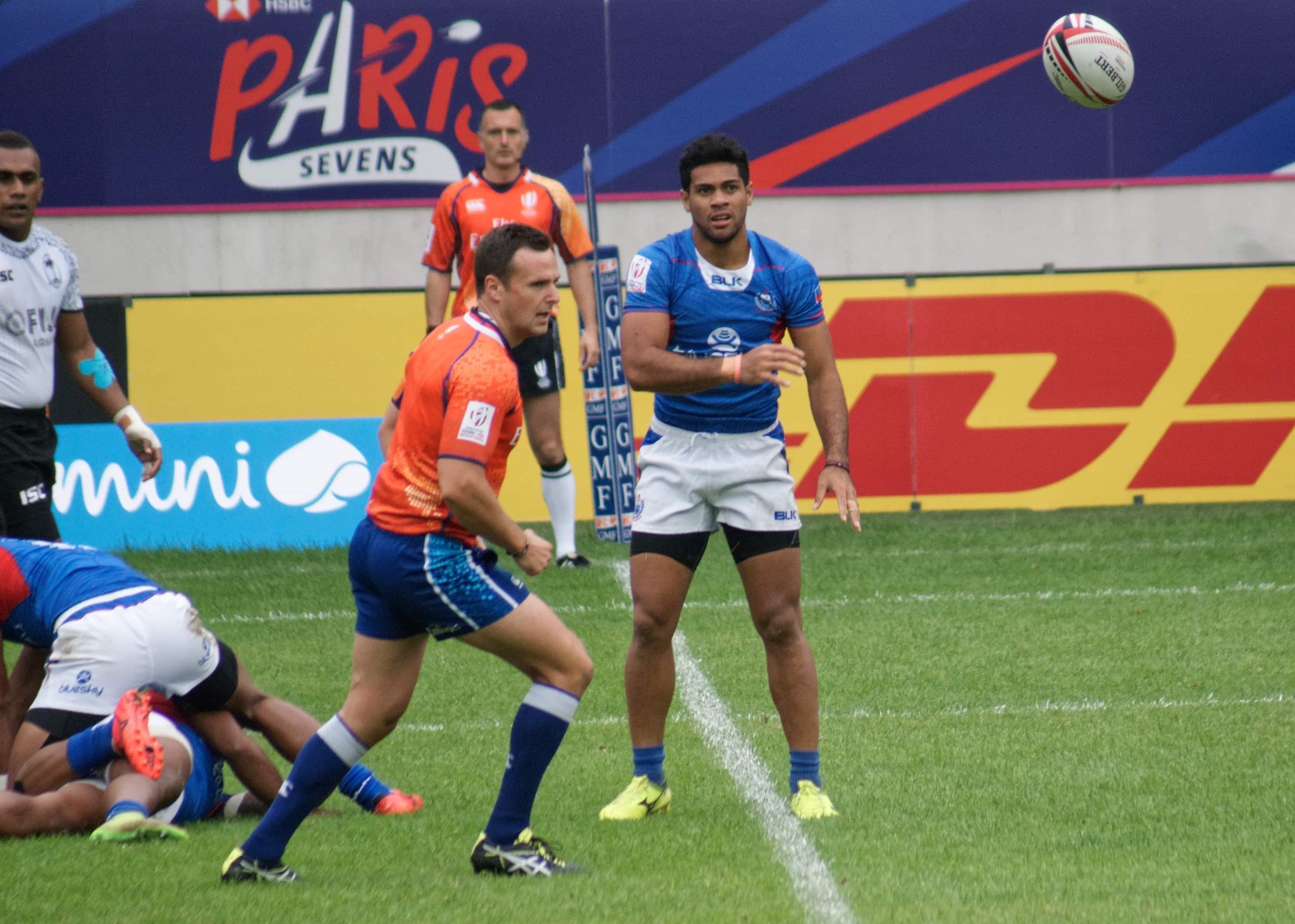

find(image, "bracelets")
[735,353,741,384]
[824,460,851,472]
[511,532,530,556]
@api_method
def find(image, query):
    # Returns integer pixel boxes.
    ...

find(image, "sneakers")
[375,790,420,811]
[789,780,837,818]
[599,774,671,819]
[557,555,591,566]
[89,812,192,838]
[219,846,301,884]
[113,691,162,780]
[471,829,581,877]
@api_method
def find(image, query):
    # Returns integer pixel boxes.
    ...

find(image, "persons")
[0,131,162,543]
[422,97,602,565]
[594,133,864,823]
[0,536,423,841]
[218,223,595,886]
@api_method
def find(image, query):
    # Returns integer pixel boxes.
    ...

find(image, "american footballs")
[1042,13,1134,109]
